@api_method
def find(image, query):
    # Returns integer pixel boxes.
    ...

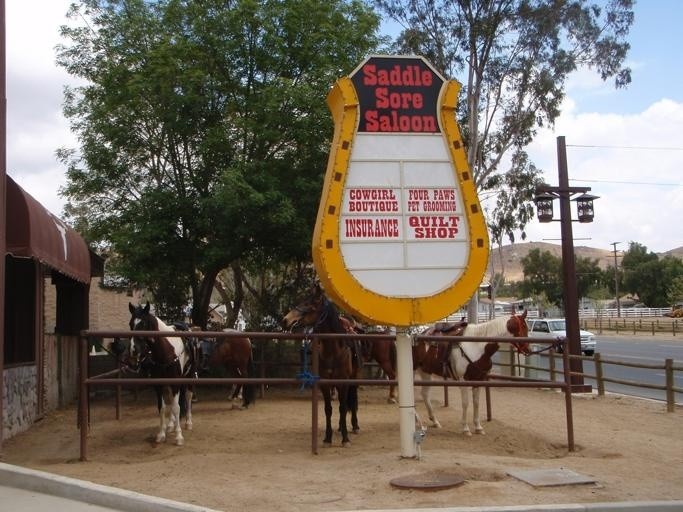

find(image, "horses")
[300,328,397,404]
[126,299,201,446]
[191,328,257,411]
[281,281,362,449]
[413,304,533,438]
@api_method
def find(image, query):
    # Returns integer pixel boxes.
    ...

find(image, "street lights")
[534,186,600,391]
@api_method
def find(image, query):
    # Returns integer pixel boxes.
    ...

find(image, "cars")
[525,317,598,357]
[666,304,682,318]
